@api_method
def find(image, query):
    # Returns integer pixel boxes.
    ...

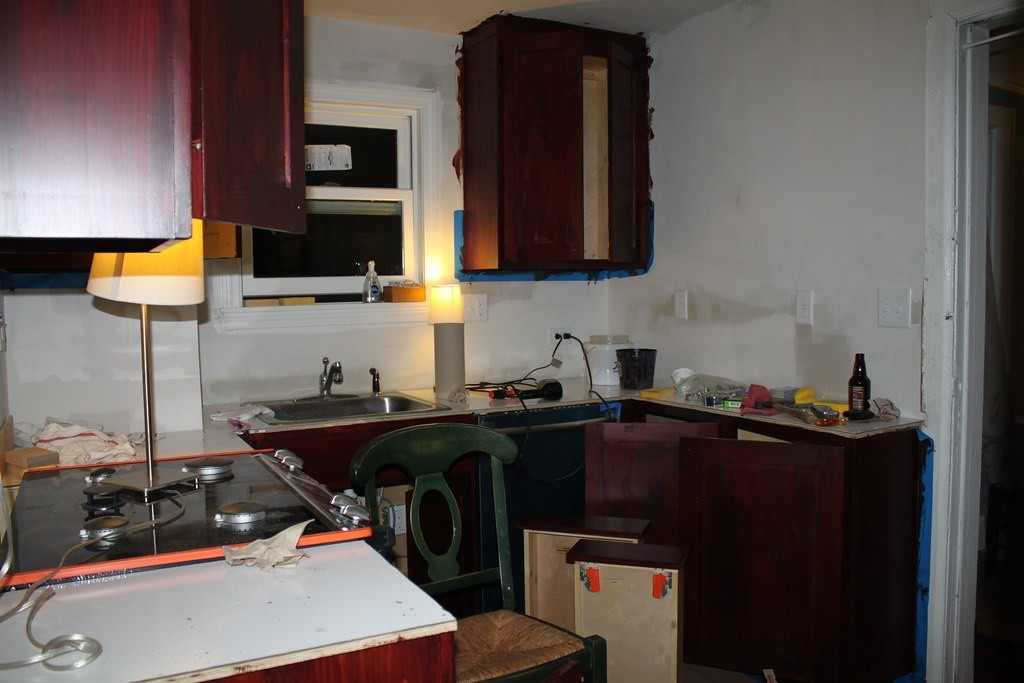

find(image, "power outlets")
[797,287,813,325]
[548,327,573,345]
[675,290,688,320]
[462,294,489,321]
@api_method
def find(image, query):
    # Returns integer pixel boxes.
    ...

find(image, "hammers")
[755,400,817,424]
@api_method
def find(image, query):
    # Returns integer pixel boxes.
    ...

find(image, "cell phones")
[810,405,840,419]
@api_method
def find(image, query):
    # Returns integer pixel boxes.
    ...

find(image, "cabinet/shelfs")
[246,413,480,615]
[453,14,654,283]
[584,400,926,683]
[0,0,307,254]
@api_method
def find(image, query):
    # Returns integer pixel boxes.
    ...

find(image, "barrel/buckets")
[581,341,634,386]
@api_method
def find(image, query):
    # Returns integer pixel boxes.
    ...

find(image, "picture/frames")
[384,285,426,302]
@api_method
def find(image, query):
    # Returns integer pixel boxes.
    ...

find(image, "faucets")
[316,356,345,402]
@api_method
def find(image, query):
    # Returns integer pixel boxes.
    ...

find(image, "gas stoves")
[0,449,374,592]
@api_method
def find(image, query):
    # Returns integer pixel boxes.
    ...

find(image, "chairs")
[351,424,608,683]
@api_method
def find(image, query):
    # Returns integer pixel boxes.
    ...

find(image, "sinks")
[239,390,454,425]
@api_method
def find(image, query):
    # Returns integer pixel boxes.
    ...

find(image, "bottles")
[848,353,871,412]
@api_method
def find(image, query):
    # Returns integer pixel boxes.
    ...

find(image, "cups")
[615,348,658,391]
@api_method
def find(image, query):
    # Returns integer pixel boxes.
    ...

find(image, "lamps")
[87,218,204,463]
[428,285,465,325]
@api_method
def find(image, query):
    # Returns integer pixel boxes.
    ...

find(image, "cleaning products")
[362,259,384,306]
